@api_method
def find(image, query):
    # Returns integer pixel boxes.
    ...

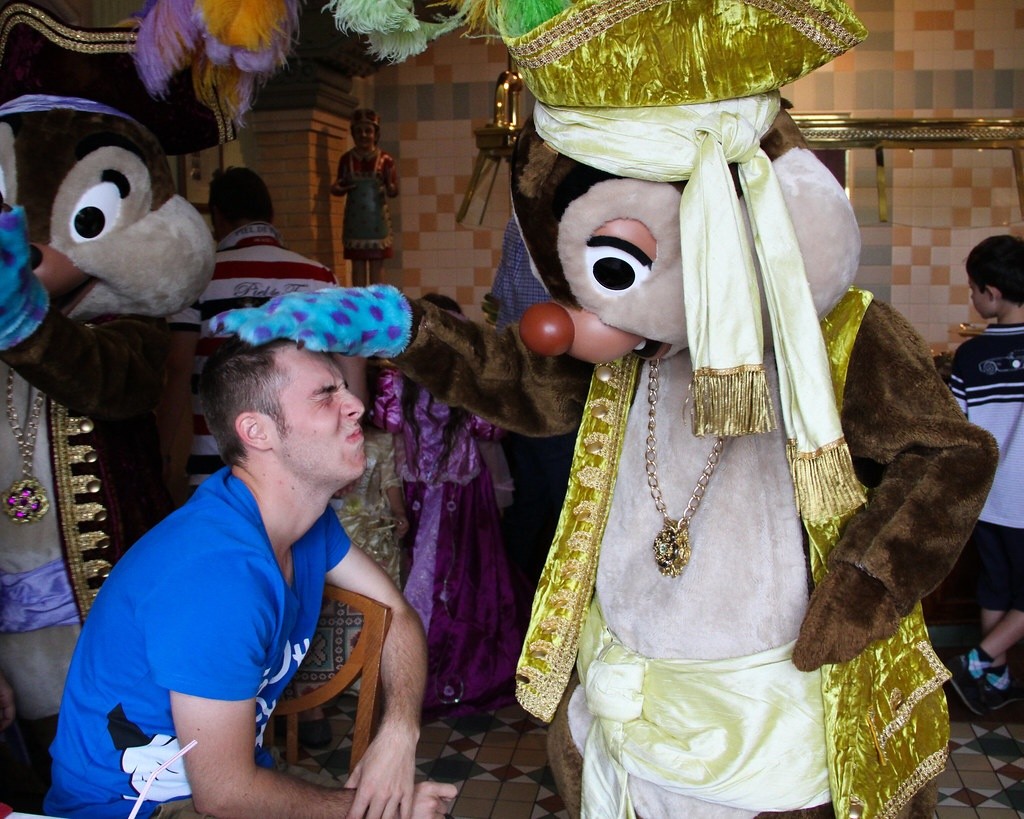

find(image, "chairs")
[266,583,394,786]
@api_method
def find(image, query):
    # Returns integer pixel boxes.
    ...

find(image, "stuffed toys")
[210,0,998,818]
[0,0,302,720]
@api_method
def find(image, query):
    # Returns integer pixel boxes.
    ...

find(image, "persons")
[370,292,525,721]
[490,215,578,586]
[944,235,1024,715]
[330,109,399,287]
[46,336,458,819]
[157,166,368,748]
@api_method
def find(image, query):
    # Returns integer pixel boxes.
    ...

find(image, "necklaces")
[0,367,50,525]
[645,358,727,578]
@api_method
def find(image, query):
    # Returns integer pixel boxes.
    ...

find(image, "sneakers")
[945,649,988,715]
[980,666,1024,709]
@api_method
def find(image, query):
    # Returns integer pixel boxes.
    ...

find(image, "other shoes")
[298,707,334,750]
